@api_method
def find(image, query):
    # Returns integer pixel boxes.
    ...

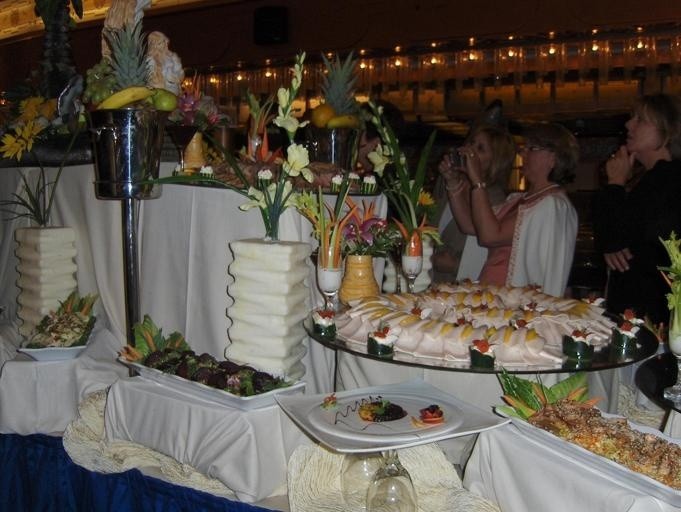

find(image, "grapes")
[82,57,115,105]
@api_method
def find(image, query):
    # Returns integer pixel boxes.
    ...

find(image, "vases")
[228,240,317,383]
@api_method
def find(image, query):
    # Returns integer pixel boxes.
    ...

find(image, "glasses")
[518,144,544,155]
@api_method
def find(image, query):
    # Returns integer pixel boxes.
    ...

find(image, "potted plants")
[86,21,158,200]
[0,119,82,338]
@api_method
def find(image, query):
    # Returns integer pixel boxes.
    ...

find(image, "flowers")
[356,92,446,246]
[179,50,314,248]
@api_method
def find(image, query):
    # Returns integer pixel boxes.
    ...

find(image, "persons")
[602,92,681,326]
[143,31,186,93]
[425,126,515,281]
[441,123,581,295]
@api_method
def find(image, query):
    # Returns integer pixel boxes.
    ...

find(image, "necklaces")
[522,181,559,201]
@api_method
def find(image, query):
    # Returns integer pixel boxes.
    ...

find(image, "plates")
[16,314,96,361]
[494,393,681,501]
[274,380,512,454]
[116,345,308,412]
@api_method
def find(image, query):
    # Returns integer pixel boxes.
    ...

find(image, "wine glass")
[391,229,424,297]
[663,303,681,402]
[339,450,419,511]
[316,245,345,315]
[166,126,198,178]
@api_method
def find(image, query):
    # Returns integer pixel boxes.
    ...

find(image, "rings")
[470,153,474,157]
[611,154,615,158]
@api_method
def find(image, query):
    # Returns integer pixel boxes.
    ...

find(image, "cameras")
[449,152,467,166]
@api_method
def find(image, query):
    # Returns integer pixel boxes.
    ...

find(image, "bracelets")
[447,184,465,200]
[469,180,487,191]
[445,179,463,192]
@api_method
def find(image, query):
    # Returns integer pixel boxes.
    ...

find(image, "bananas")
[96,86,153,109]
[327,115,359,127]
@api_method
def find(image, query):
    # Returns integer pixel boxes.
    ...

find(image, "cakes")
[173,157,377,196]
[313,279,645,372]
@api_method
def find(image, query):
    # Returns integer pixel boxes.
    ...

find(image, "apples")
[144,88,172,103]
[154,94,178,111]
